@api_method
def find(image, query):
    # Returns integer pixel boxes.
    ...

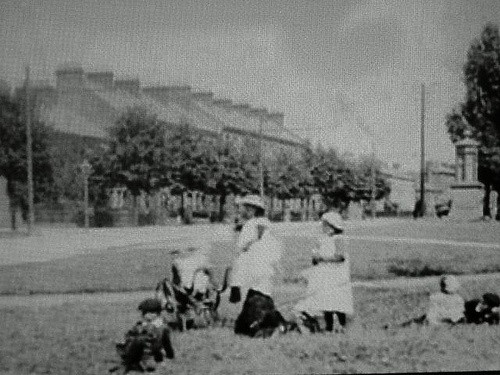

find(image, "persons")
[115,298,175,375]
[292,210,355,334]
[222,195,281,328]
[424,273,467,331]
[384,293,499,332]
[167,244,210,304]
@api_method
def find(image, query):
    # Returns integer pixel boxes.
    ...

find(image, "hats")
[322,212,344,231]
[234,194,267,211]
[138,299,160,311]
